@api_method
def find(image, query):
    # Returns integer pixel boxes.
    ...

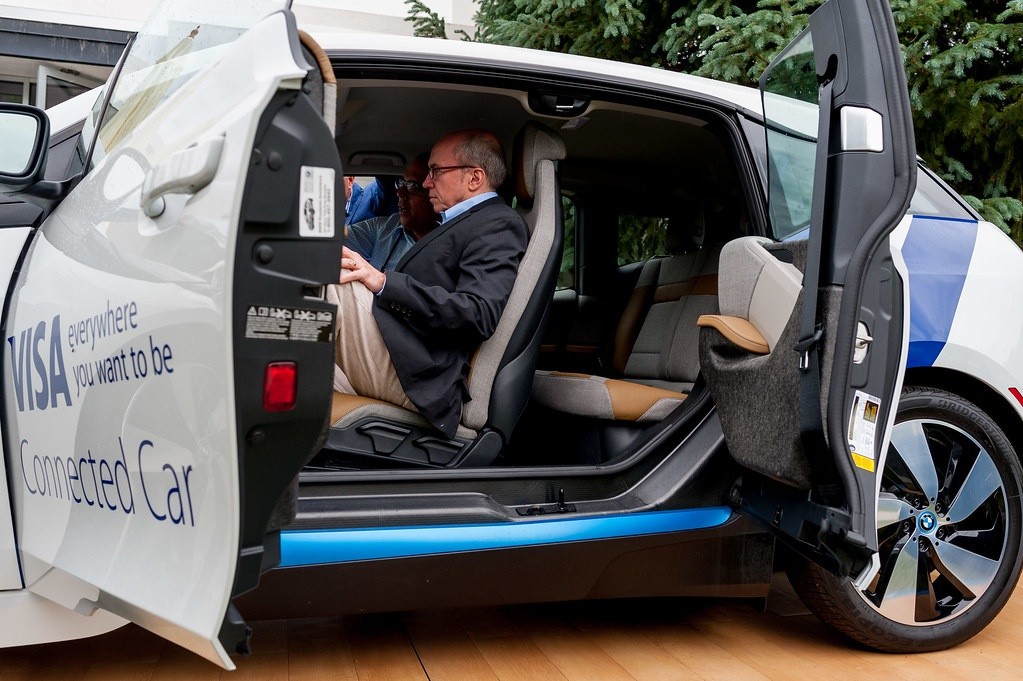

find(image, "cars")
[1,0,1023,676]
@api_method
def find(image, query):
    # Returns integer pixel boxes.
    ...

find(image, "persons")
[326,130,531,439]
[342,175,391,226]
[344,151,442,272]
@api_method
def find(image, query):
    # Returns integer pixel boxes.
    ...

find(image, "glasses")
[428,165,476,180]
[395,178,423,194]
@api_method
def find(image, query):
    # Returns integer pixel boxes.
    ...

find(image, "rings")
[350,260,357,269]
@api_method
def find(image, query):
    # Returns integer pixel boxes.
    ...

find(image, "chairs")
[324,119,567,470]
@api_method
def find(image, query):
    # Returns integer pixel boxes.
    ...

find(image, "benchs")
[528,254,720,422]
